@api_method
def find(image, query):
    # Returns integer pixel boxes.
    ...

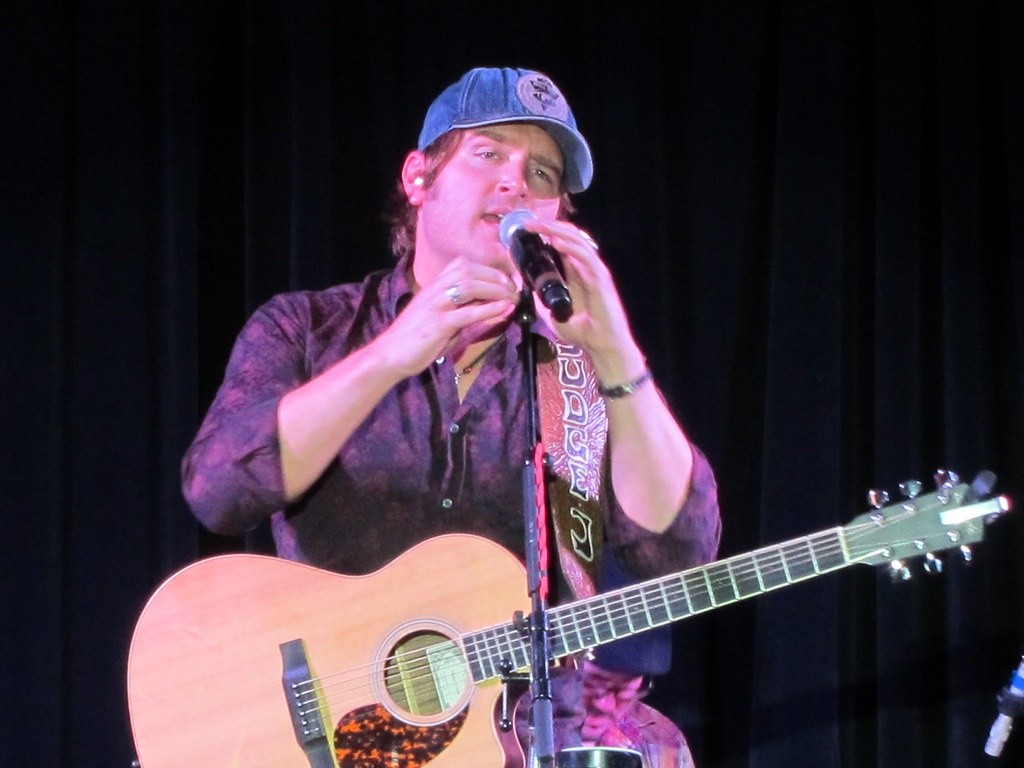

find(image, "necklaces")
[449,323,510,388]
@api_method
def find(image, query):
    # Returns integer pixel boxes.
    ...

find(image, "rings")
[447,282,461,306]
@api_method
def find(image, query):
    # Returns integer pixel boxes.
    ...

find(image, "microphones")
[498,209,573,324]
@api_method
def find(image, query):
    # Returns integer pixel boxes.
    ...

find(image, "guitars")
[124,468,1014,768]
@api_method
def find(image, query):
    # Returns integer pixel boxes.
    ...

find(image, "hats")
[417,65,595,196]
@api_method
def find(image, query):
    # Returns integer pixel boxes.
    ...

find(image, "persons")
[576,547,699,767]
[179,68,723,754]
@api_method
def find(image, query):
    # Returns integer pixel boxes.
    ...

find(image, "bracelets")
[594,365,652,400]
